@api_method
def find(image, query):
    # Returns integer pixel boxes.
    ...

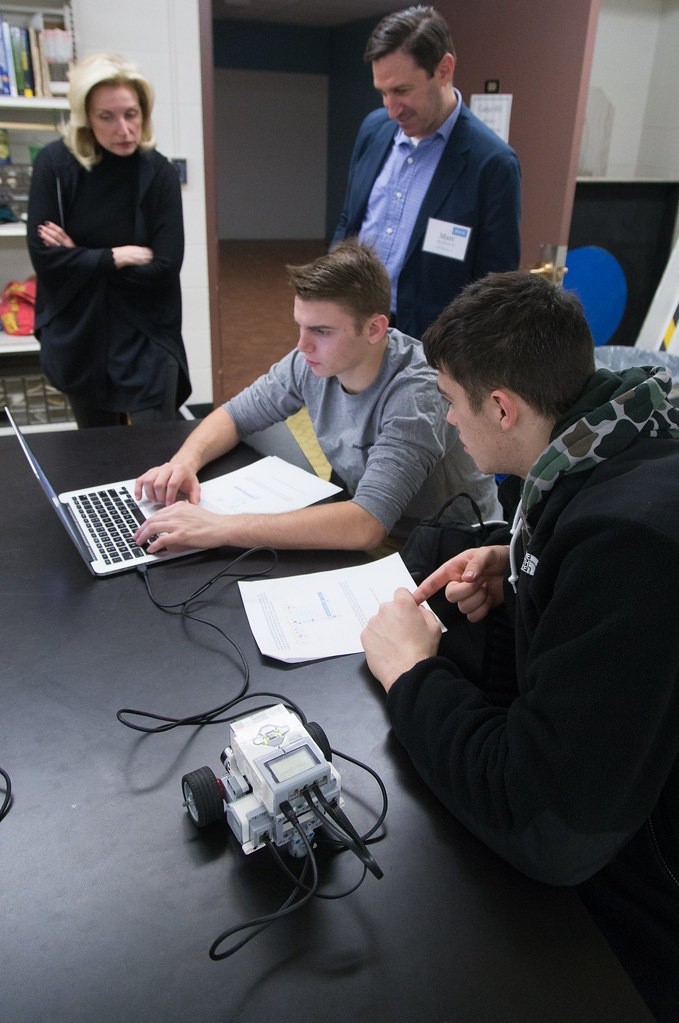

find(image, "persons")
[134,237,509,553]
[365,271,679,1023]
[326,3,522,344]
[27,55,191,433]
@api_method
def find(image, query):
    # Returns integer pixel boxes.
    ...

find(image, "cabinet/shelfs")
[0,0,79,431]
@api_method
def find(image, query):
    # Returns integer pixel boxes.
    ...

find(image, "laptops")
[4,405,209,579]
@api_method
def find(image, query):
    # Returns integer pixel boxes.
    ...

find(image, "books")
[0,12,75,99]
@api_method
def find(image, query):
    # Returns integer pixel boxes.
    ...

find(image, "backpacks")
[399,492,513,622]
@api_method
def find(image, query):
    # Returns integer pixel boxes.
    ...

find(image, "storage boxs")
[0,374,75,427]
[0,165,33,221]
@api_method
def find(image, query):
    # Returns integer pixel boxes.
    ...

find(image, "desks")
[0,420,656,1023]
